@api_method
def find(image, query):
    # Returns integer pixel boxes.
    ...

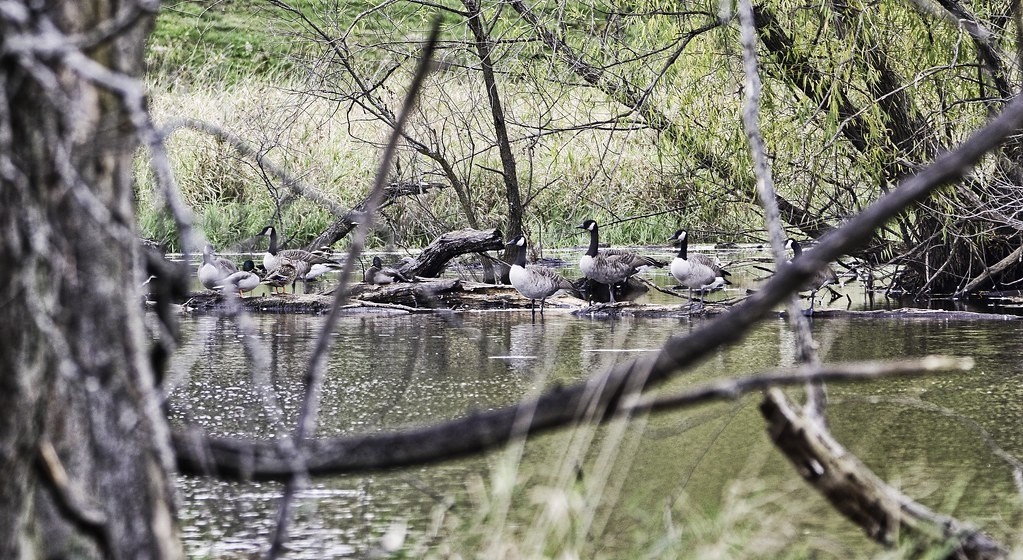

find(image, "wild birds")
[783,238,839,317]
[508,235,578,317]
[196,226,410,299]
[575,219,669,305]
[666,230,732,307]
